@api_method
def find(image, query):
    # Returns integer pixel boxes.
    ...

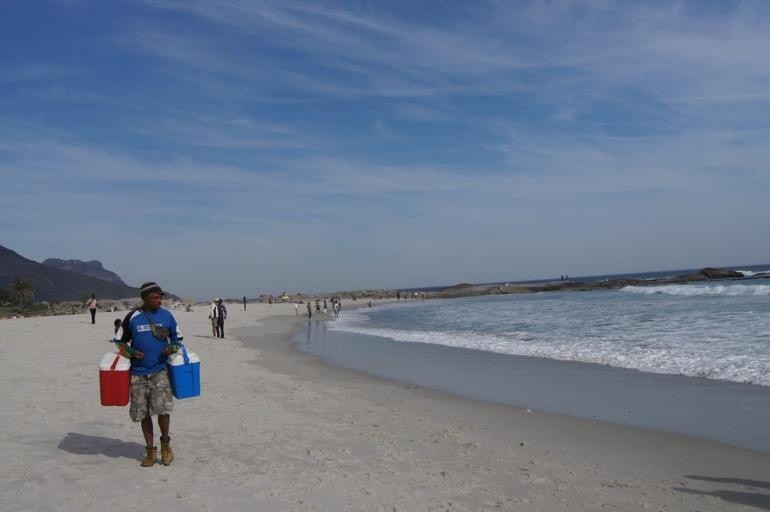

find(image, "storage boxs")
[98,343,132,406]
[166,341,203,401]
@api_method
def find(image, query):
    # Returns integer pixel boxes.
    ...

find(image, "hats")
[140,282,165,300]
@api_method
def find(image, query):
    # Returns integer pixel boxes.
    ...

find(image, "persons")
[292,297,340,319]
[186,304,191,312]
[216,299,227,338]
[269,294,273,308]
[243,295,247,312]
[113,318,125,343]
[86,293,98,325]
[209,296,219,336]
[112,281,184,467]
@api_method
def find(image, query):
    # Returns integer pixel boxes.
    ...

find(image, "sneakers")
[160,435,173,466]
[142,446,157,467]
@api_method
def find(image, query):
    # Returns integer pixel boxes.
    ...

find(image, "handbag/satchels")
[149,321,169,341]
